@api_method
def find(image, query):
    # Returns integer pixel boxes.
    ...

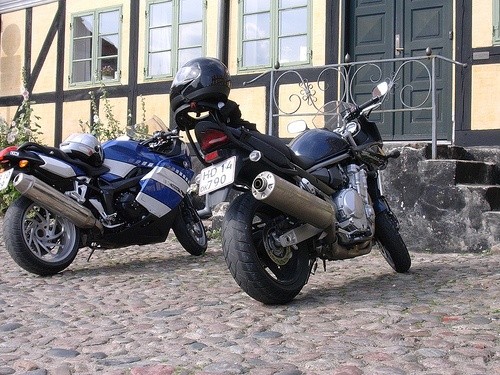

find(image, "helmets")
[170,56,231,111]
[60,132,106,168]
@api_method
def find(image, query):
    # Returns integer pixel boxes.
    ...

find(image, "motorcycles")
[0,114,212,277]
[180,81,412,306]
